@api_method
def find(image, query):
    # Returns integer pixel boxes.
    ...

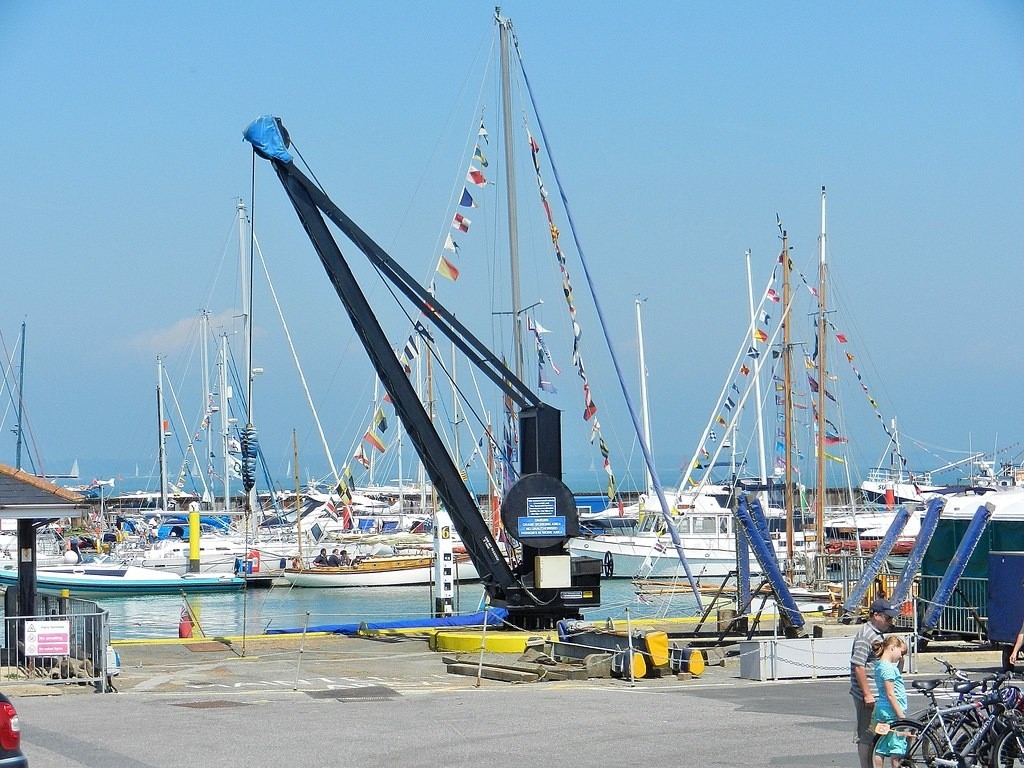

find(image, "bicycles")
[869,663,1024,768]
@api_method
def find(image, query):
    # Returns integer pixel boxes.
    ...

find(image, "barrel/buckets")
[247,559,253,574]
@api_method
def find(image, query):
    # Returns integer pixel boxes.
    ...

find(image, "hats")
[871,599,899,617]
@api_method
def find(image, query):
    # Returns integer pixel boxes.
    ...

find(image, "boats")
[823,432,1024,561]
[0,199,527,594]
[303,5,659,547]
[562,184,926,569]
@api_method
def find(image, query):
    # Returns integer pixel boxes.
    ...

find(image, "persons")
[147,514,161,543]
[655,518,665,535]
[849,599,899,768]
[313,549,349,568]
[1010,622,1024,664]
[872,636,908,768]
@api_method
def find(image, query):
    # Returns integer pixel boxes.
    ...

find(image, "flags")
[527,124,623,521]
[322,124,491,523]
[646,254,920,570]
[179,392,214,488]
